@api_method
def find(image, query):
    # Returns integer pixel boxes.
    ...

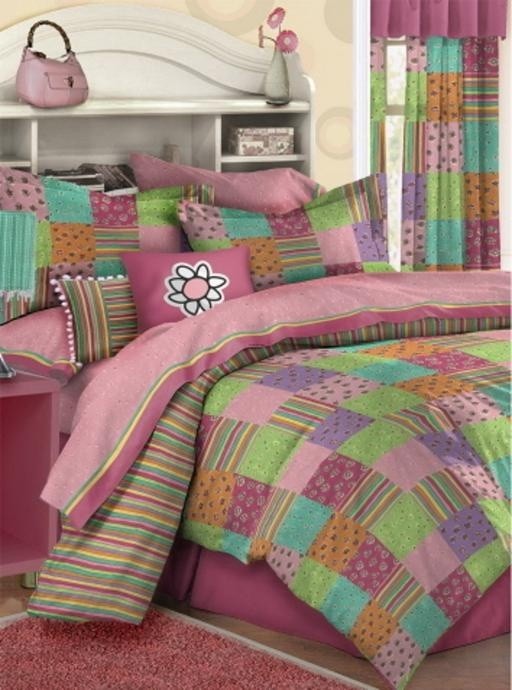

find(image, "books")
[37,163,139,197]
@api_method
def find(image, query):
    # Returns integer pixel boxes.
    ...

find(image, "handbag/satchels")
[18,19,88,110]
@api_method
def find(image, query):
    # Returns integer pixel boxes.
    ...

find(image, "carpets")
[0,600,382,690]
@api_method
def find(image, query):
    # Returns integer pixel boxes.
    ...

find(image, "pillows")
[131,153,326,216]
[116,246,257,335]
[182,171,393,297]
[2,162,185,327]
[51,271,139,365]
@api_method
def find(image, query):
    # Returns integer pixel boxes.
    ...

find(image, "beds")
[0,4,511,659]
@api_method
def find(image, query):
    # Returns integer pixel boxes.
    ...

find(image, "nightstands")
[0,378,58,585]
